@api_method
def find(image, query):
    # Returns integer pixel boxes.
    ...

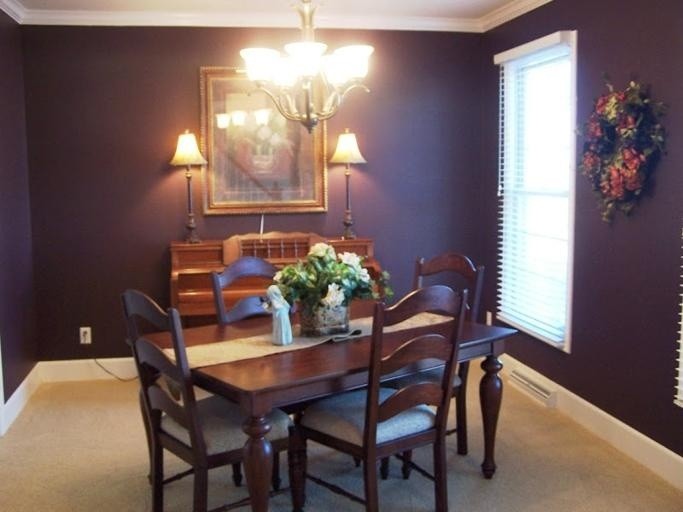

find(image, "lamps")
[329,126,368,240]
[235,1,376,136]
[169,128,210,245]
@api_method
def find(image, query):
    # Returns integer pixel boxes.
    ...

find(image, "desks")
[137,304,520,512]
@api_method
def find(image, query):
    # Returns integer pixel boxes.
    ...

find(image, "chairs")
[210,256,293,322]
[379,253,487,480]
[121,289,294,512]
[291,285,468,512]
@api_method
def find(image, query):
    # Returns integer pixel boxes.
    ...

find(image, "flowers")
[260,242,393,311]
[570,69,671,224]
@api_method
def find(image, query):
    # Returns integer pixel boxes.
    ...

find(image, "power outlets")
[78,327,92,345]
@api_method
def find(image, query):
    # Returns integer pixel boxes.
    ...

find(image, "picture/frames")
[198,64,329,217]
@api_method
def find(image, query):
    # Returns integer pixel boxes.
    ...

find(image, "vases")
[299,299,350,338]
